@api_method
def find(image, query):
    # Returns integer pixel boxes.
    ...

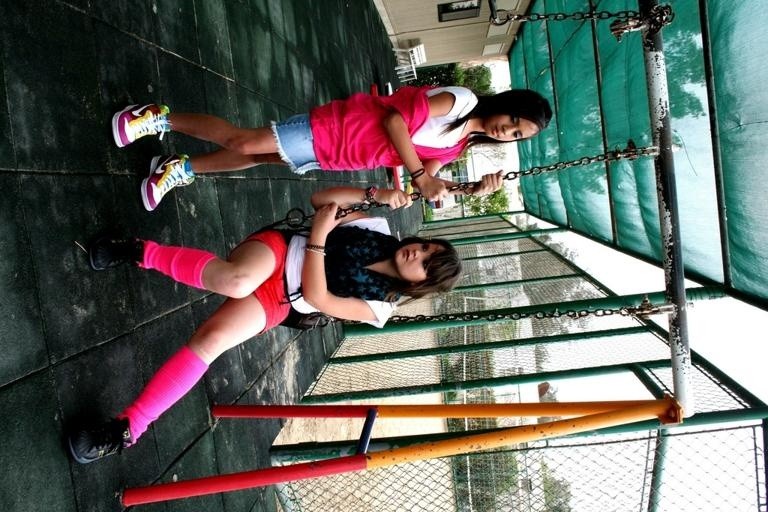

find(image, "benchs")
[391,42,429,84]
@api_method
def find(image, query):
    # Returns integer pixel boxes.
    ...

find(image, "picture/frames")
[437,1,482,22]
